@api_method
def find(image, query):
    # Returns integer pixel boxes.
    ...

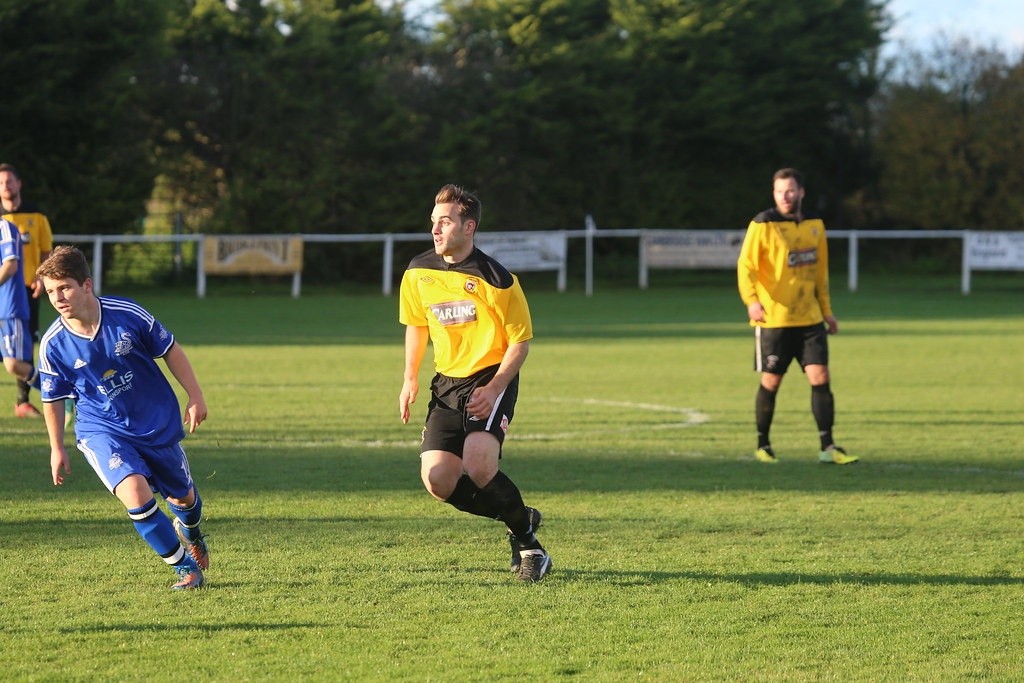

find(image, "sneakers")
[170,557,205,590]
[173,517,209,571]
[63,393,74,429]
[819,446,859,464]
[516,538,552,583]
[755,447,779,463]
[15,401,42,419]
[506,506,542,573]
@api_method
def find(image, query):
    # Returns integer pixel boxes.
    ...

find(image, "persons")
[737,168,861,466]
[0,219,77,428]
[0,164,52,418]
[36,245,208,591]
[399,185,552,582]
